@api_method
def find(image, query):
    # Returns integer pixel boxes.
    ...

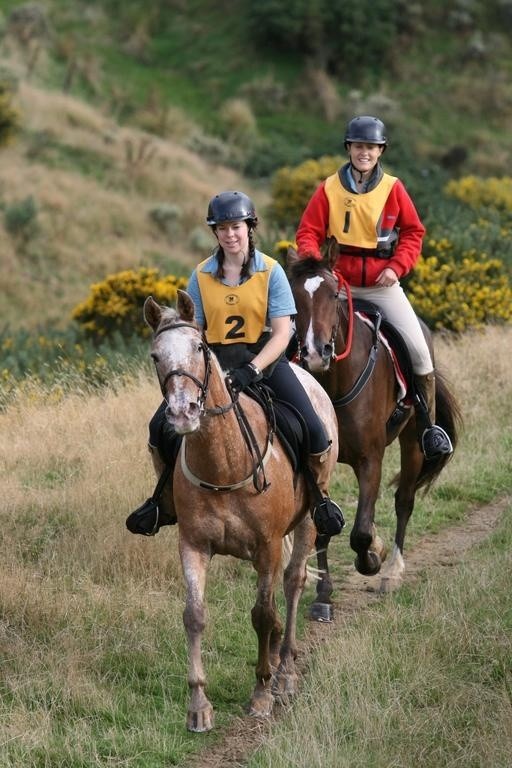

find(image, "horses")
[286,235,465,622]
[144,288,340,731]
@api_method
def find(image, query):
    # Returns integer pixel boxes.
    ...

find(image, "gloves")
[228,362,259,393]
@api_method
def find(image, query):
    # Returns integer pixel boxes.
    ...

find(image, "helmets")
[208,191,258,225]
[344,116,389,146]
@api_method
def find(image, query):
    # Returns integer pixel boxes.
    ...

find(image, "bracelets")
[249,363,260,376]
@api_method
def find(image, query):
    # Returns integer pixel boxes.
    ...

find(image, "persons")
[125,190,344,536]
[294,117,451,462]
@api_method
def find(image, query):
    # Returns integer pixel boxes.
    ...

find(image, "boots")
[305,444,343,536]
[127,442,177,534]
[413,370,450,459]
